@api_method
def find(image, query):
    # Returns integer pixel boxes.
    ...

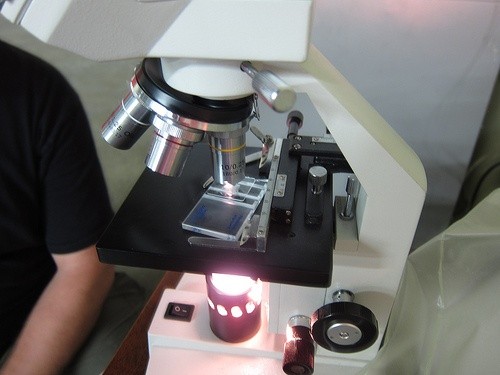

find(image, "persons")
[0,40,114,375]
